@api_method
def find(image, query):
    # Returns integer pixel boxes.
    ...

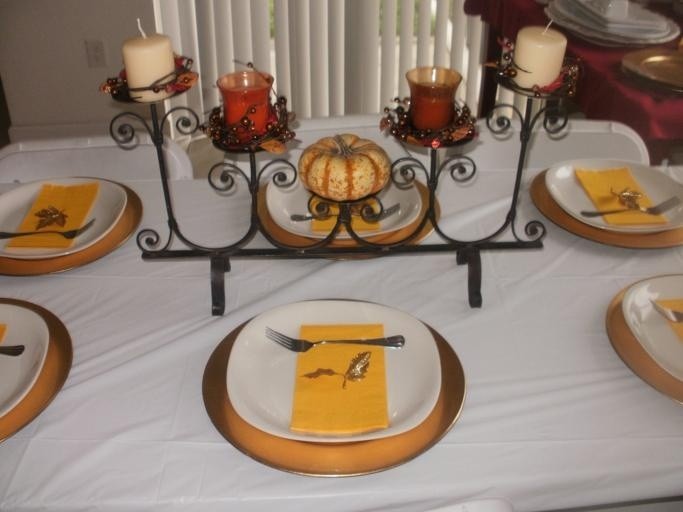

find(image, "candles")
[513,17,566,89]
[122,18,175,101]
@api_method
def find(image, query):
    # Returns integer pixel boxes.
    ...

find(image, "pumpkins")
[297,134,392,202]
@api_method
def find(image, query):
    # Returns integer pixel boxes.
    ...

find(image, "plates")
[0,296,74,445]
[621,275,683,383]
[225,297,445,448]
[543,157,683,234]
[604,274,683,407]
[251,166,441,250]
[616,46,683,97]
[265,167,424,244]
[0,174,144,277]
[0,177,127,261]
[543,0,681,49]
[199,294,469,482]
[529,164,683,249]
[0,303,49,420]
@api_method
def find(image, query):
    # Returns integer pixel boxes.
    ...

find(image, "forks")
[0,216,96,239]
[260,322,406,354]
[580,194,681,219]
[647,297,683,324]
[289,201,401,223]
